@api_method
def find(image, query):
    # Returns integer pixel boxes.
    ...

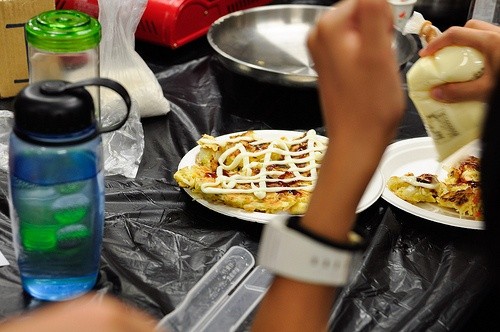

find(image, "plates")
[380,136,486,230]
[177,129,385,225]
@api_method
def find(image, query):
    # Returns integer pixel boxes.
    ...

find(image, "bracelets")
[258,211,363,290]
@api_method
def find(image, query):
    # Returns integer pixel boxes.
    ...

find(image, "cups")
[23,8,102,128]
[7,77,131,302]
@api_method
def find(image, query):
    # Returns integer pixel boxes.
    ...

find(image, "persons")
[248,0,500,332]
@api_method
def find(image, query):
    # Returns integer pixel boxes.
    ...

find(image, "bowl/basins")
[207,4,418,90]
